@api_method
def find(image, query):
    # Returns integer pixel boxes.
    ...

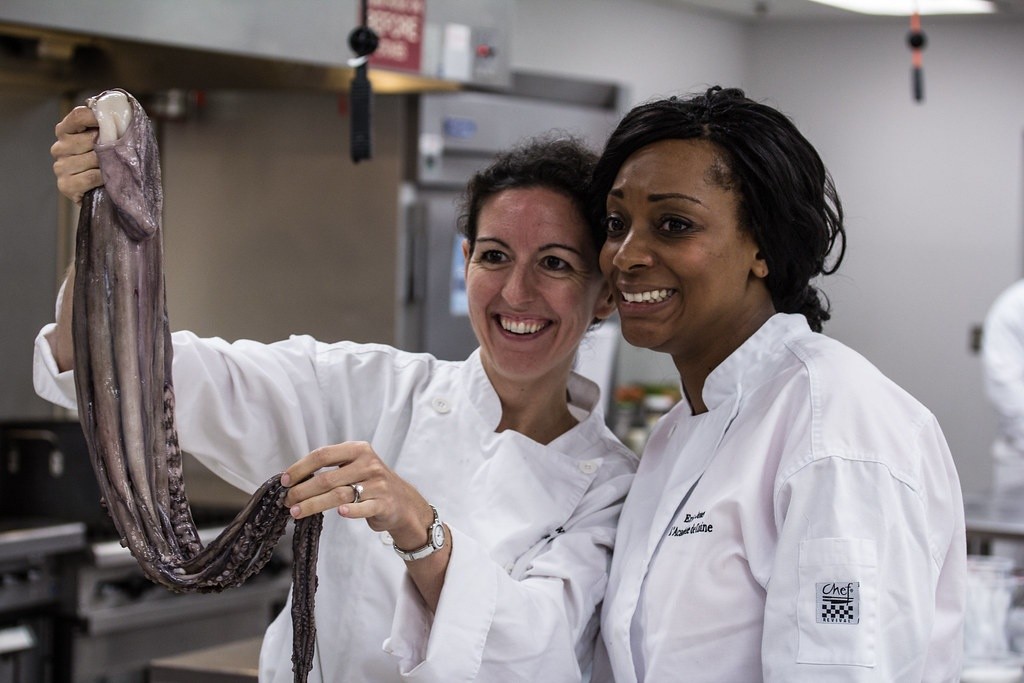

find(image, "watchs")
[392,502,446,562]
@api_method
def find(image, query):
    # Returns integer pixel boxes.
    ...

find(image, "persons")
[976,276,1023,565]
[32,105,642,683]
[589,83,968,683]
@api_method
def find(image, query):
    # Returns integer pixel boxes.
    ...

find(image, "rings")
[349,481,364,504]
